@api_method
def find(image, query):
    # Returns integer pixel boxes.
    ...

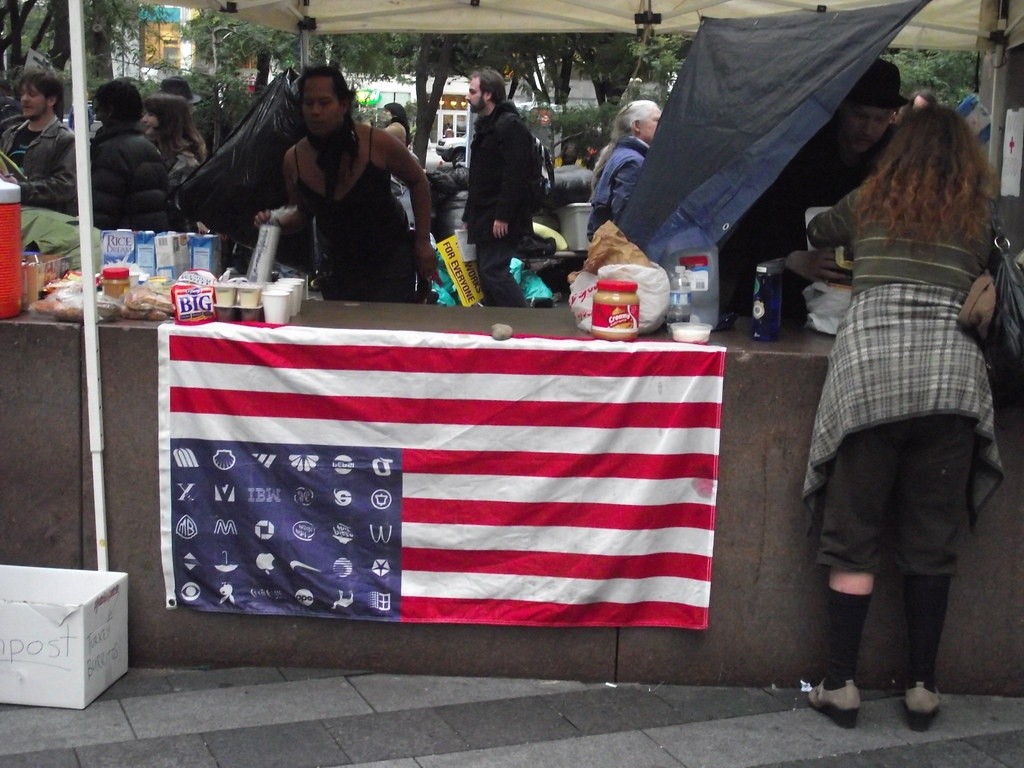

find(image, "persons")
[139,93,211,235]
[0,68,78,217]
[91,78,174,235]
[462,66,545,309]
[797,102,1008,731]
[587,100,662,244]
[445,123,454,138]
[68,91,93,130]
[0,78,28,159]
[378,101,411,196]
[772,55,912,288]
[253,66,440,305]
[911,89,937,113]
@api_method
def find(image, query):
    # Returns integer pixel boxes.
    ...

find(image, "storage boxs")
[0,564,129,711]
[99,230,222,279]
[532,202,593,251]
[21,254,74,311]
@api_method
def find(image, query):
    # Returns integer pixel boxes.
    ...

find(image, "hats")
[842,59,909,108]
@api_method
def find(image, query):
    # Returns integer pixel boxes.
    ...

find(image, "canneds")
[102,267,130,299]
[591,279,640,342]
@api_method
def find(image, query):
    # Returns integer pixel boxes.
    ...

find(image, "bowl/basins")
[671,323,712,343]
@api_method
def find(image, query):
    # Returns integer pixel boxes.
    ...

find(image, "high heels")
[902,682,940,730]
[805,679,860,729]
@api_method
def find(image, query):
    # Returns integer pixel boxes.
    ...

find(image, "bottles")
[587,279,640,340]
[666,266,691,333]
[103,268,130,297]
[751,266,782,341]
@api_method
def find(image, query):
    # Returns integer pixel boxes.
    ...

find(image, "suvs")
[435,129,475,167]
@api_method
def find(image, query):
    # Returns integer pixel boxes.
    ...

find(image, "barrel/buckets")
[0,179,21,318]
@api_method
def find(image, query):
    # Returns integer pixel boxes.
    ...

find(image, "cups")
[261,278,305,324]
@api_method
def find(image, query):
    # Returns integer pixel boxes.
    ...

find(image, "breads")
[33,282,176,323]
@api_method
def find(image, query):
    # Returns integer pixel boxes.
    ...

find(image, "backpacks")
[494,112,556,209]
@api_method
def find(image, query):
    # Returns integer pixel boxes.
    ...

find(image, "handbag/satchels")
[569,260,669,334]
[984,246,1024,409]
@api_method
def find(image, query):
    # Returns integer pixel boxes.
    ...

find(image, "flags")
[158,321,726,628]
